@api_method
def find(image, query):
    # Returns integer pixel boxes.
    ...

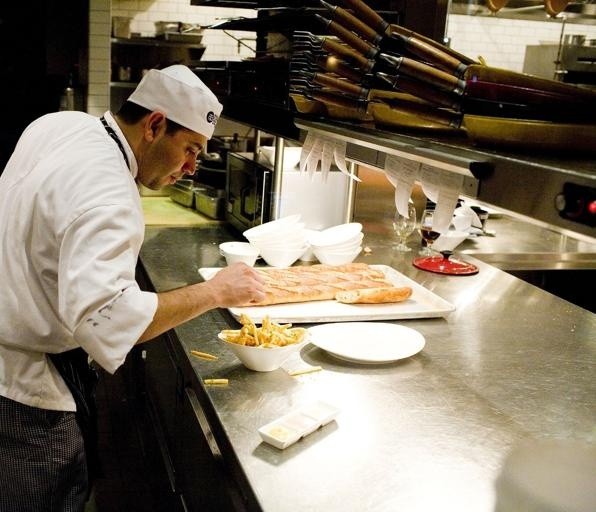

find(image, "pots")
[306,2,595,158]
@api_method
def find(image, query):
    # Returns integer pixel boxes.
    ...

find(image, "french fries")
[190,313,322,384]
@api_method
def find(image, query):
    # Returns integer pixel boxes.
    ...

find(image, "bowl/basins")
[430,229,470,252]
[219,212,366,269]
[216,327,313,374]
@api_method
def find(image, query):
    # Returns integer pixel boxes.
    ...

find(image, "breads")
[238,262,413,305]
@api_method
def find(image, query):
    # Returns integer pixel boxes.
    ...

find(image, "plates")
[308,319,427,369]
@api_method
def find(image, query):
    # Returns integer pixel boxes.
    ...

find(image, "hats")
[127,64,224,140]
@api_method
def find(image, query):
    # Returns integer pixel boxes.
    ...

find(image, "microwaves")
[222,142,355,239]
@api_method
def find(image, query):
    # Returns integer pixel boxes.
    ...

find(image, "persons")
[0,61,270,511]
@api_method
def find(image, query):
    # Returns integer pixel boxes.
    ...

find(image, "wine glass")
[418,207,444,259]
[391,199,418,253]
